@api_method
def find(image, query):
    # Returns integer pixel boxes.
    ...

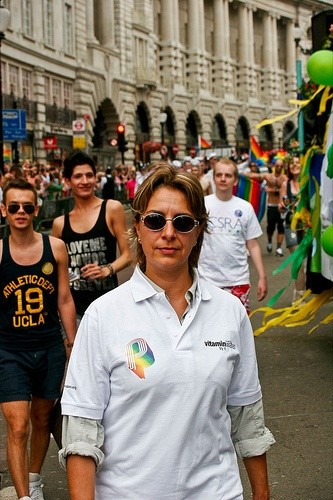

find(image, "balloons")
[307,49,333,88]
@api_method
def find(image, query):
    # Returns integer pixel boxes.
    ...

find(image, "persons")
[50,148,134,361]
[58,162,278,500]
[0,148,312,309]
[0,179,78,500]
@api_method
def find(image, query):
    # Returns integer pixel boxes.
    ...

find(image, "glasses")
[4,204,36,215]
[140,212,202,234]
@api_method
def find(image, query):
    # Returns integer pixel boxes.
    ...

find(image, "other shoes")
[276,248,282,255]
[265,241,273,253]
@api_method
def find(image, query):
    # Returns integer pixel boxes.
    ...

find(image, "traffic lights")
[116,122,128,152]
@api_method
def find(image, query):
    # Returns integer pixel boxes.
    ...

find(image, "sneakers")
[20,472,45,500]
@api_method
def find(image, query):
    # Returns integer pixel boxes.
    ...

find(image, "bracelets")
[101,265,114,276]
[67,343,73,348]
